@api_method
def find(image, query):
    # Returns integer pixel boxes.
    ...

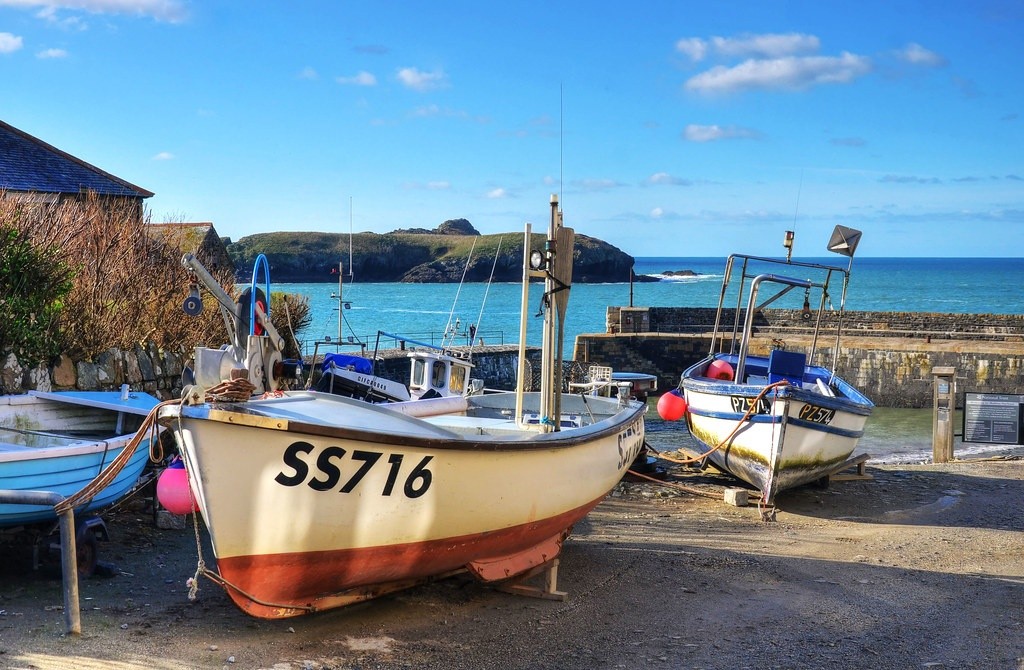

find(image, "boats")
[152,194,654,620]
[679,227,876,508]
[317,229,505,410]
[1,385,174,535]
